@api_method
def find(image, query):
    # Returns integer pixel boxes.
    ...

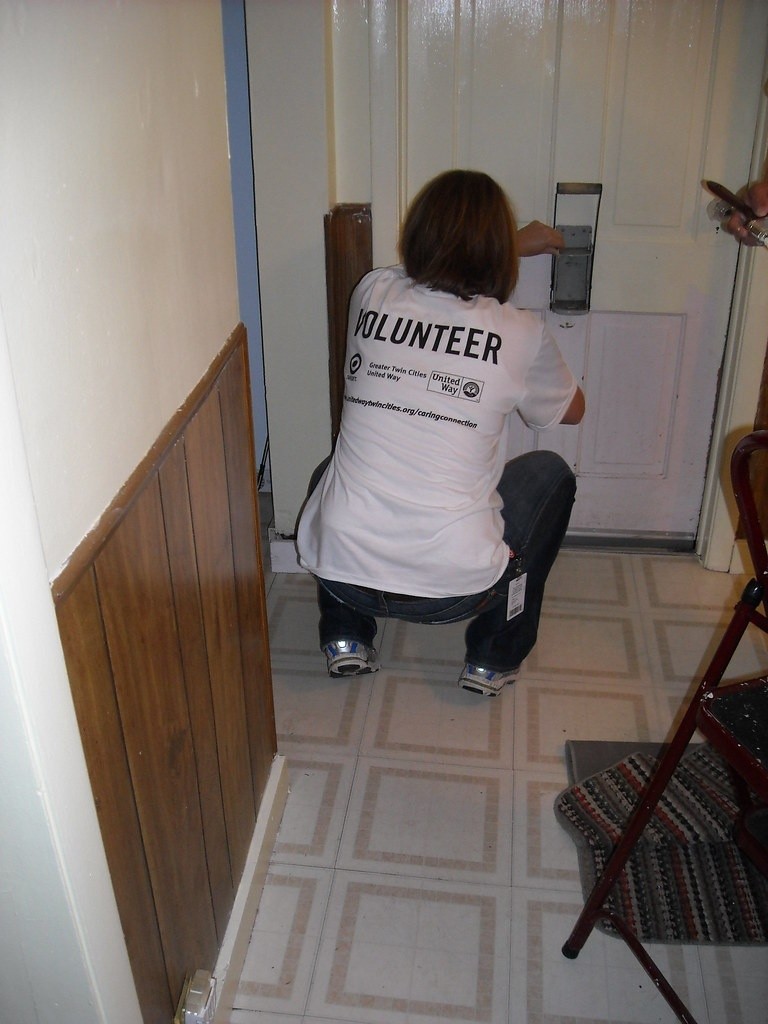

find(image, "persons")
[726,176,768,246]
[290,168,587,699]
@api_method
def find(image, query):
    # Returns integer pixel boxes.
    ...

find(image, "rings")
[735,226,742,234]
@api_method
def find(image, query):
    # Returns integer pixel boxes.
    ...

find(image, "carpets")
[552,740,768,948]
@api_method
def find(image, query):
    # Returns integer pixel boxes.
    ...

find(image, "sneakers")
[457,662,520,697]
[323,640,379,677]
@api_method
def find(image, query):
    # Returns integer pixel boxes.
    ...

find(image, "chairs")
[560,428,768,1022]
